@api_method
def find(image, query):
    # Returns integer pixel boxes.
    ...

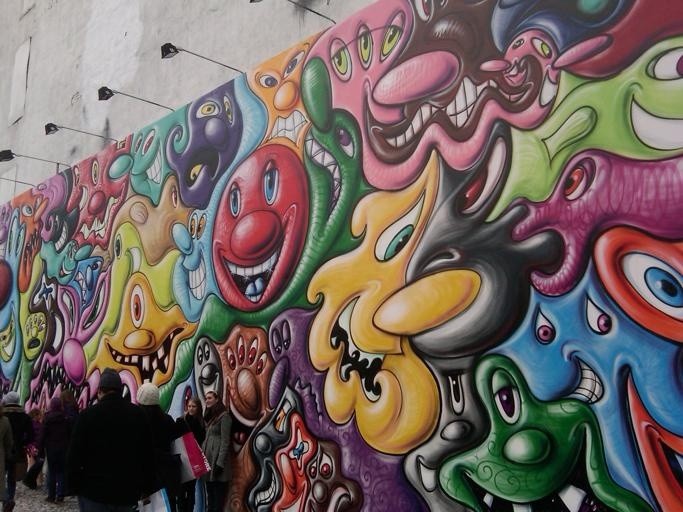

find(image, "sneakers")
[4,475,64,512]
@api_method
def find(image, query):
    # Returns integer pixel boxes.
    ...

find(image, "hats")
[2,392,20,405]
[136,382,161,405]
[99,367,123,389]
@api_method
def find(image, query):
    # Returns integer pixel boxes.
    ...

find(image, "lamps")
[0,43,244,167]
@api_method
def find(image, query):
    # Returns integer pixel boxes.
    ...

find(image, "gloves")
[212,464,224,480]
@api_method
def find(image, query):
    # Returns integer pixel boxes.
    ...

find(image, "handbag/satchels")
[168,432,212,484]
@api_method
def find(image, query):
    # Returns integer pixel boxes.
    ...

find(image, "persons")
[0,367,232,511]
